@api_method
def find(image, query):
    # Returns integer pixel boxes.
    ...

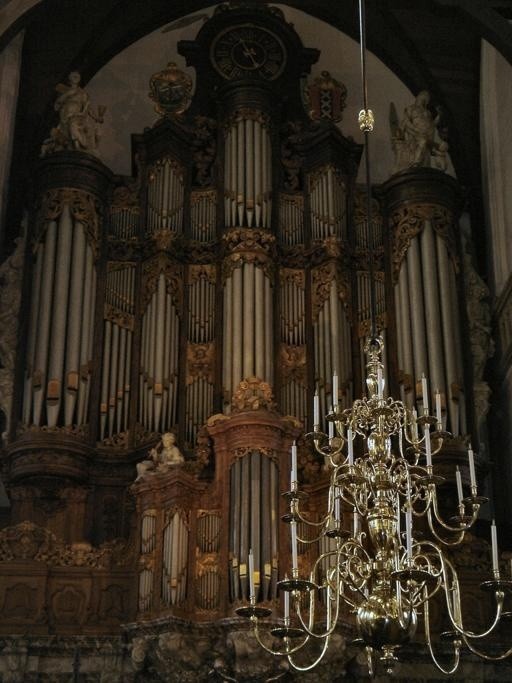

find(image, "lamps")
[234,0,512,677]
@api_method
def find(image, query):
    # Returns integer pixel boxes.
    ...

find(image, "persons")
[136,432,185,479]
[399,90,443,167]
[53,71,91,150]
[321,436,347,472]
